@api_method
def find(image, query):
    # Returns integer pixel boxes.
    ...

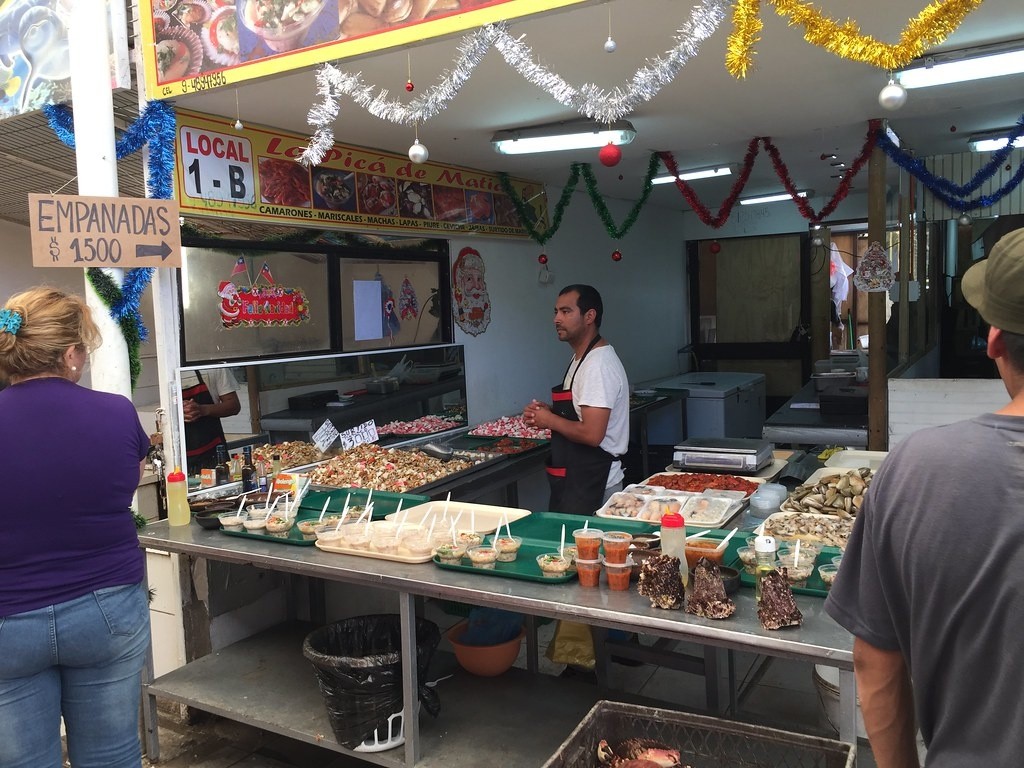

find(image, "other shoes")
[604,629,643,667]
[559,662,597,686]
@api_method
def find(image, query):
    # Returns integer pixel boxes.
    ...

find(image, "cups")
[315,170,354,211]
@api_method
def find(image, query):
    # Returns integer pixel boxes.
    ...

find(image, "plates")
[152,0,239,84]
[259,158,310,207]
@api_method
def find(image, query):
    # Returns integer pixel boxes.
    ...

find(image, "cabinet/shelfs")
[133,387,854,768]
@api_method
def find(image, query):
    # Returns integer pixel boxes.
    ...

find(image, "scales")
[673,438,774,477]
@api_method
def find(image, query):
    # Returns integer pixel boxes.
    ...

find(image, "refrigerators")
[650,371,767,438]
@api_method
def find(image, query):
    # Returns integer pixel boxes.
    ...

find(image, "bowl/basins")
[689,565,741,594]
[466,545,499,570]
[435,542,467,565]
[536,544,578,577]
[489,535,522,562]
[457,532,486,558]
[736,536,843,590]
[447,622,525,676]
[239,0,324,51]
[628,534,661,581]
[189,491,453,557]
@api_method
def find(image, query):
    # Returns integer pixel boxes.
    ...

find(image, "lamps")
[489,116,637,155]
[651,164,734,185]
[736,190,808,207]
[967,128,1024,152]
[884,39,1024,92]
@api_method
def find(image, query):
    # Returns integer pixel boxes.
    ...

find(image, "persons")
[182,368,241,475]
[823,227,1024,768]
[1,286,151,768]
[523,284,629,514]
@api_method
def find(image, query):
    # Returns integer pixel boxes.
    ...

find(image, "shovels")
[422,443,471,462]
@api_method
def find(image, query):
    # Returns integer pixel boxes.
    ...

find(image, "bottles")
[166,466,191,527]
[242,446,258,492]
[660,510,689,587]
[755,537,775,601]
[257,455,267,492]
[272,455,281,490]
[215,445,243,486]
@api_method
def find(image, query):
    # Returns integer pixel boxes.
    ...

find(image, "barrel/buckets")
[813,664,868,740]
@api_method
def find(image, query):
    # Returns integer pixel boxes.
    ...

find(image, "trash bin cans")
[302,612,441,753]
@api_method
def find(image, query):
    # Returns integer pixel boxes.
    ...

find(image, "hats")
[961,227,1024,334]
